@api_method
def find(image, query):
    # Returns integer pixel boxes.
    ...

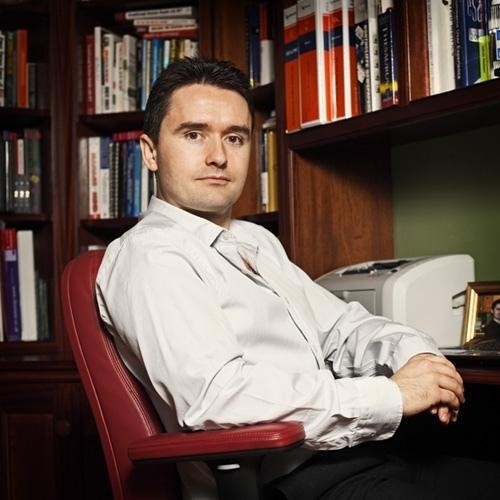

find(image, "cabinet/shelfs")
[0,0,395,373]
[273,0,499,146]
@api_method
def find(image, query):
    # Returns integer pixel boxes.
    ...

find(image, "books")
[281,0,500,133]
[79,131,157,220]
[86,7,198,115]
[1,129,42,215]
[1,223,50,340]
[247,0,281,215]
[1,29,47,109]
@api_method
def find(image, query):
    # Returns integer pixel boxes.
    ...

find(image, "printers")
[312,253,476,348]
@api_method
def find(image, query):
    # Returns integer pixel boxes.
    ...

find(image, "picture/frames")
[461,279,500,351]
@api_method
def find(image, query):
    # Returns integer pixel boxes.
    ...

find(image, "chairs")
[61,249,305,499]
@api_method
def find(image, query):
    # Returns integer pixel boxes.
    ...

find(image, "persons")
[482,297,500,342]
[93,53,500,499]
